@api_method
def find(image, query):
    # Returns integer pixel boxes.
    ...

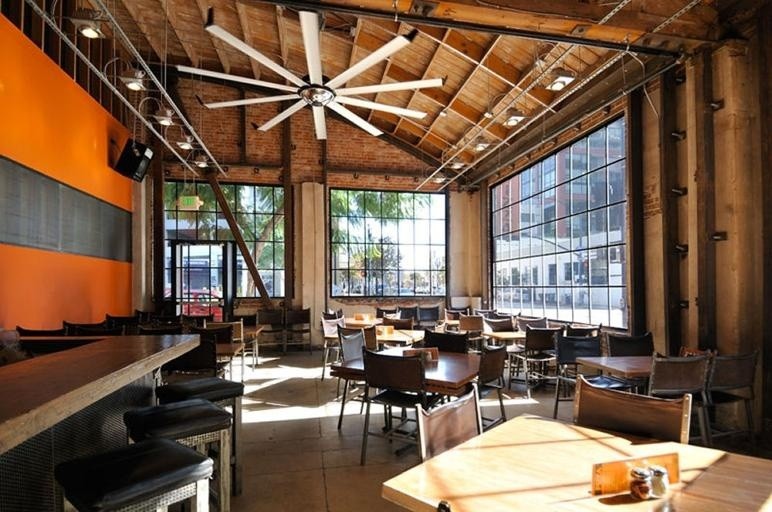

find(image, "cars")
[333,281,445,296]
[497,286,529,304]
[166,290,226,323]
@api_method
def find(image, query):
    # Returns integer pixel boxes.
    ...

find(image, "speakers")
[114,137,154,184]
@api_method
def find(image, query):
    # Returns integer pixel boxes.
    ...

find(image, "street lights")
[520,232,584,304]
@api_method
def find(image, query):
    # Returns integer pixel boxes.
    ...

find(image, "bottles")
[648,466,670,498]
[416,350,433,362]
[683,351,695,358]
[628,468,653,499]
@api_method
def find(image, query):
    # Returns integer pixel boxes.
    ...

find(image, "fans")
[173,9,446,141]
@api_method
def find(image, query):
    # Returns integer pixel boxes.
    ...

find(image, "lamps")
[50,0,107,41]
[103,56,146,92]
[533,42,573,93]
[487,94,535,125]
[422,127,490,184]
[138,97,210,170]
